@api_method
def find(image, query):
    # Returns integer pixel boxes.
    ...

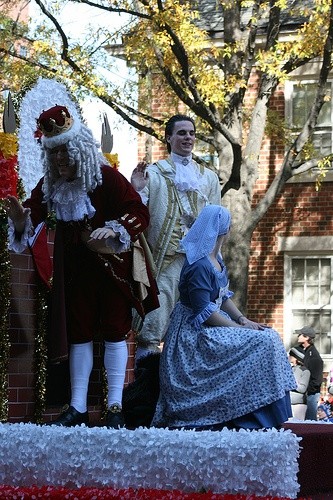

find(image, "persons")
[1,105,150,428]
[289,327,333,422]
[130,114,221,348]
[151,205,298,430]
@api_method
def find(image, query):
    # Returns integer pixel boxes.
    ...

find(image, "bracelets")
[238,315,245,322]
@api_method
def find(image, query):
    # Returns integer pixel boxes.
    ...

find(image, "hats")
[295,326,315,338]
[37,103,81,148]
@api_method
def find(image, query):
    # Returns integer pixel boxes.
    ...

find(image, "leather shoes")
[45,404,90,428]
[108,410,124,429]
